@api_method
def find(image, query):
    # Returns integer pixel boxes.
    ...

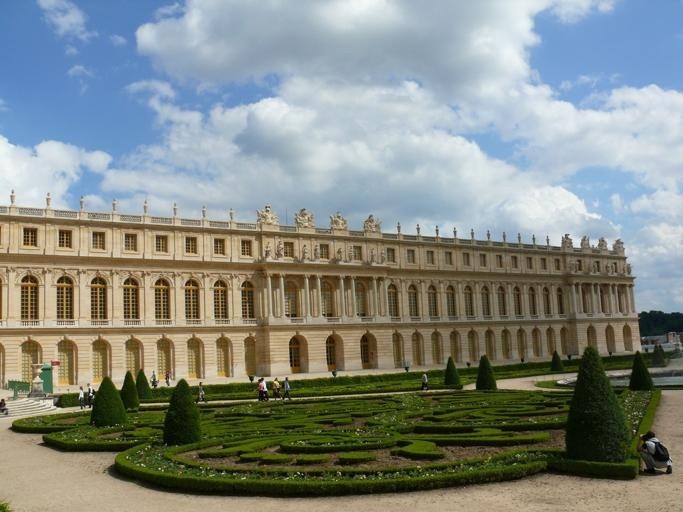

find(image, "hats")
[646,431,655,438]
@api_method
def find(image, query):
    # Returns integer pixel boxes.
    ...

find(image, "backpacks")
[646,441,669,462]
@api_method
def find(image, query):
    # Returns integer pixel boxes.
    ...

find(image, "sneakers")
[666,465,672,474]
[644,468,656,474]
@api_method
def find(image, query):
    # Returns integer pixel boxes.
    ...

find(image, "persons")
[257,376,291,401]
[637,430,673,474]
[78,382,94,409]
[0,399,5,412]
[165,370,170,386]
[196,381,206,403]
[150,370,157,388]
[421,372,429,390]
